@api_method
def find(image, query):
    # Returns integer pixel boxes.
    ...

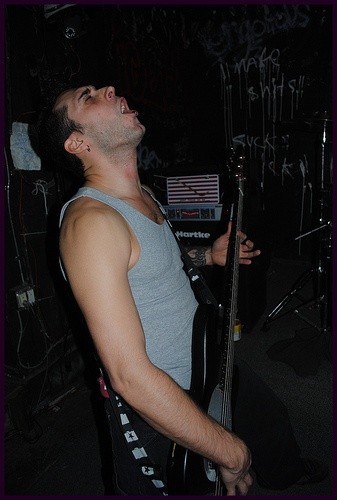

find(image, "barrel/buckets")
[233,319,242,342]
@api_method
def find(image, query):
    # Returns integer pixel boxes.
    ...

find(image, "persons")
[39,84,326,496]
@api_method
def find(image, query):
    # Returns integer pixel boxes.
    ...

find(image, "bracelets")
[194,245,208,263]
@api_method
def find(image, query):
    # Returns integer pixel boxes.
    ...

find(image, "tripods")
[262,121,333,332]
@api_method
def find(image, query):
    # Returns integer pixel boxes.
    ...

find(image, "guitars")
[169,142,251,496]
